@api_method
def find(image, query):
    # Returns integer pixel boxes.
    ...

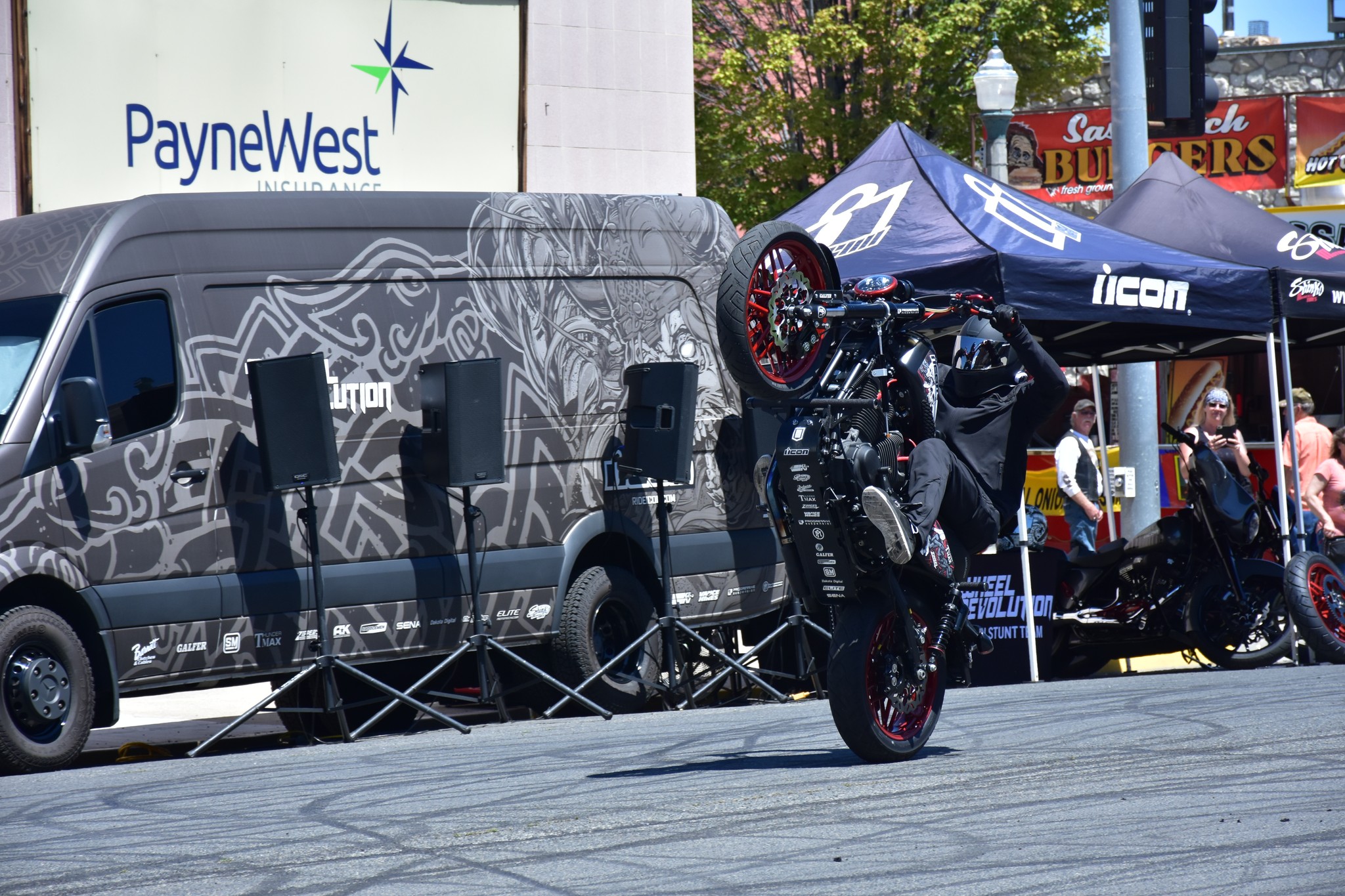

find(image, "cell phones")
[1216,426,1228,448]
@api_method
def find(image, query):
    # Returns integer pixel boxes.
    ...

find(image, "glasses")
[1079,409,1096,416]
[1208,402,1226,408]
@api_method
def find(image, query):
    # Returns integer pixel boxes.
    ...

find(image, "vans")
[1,189,794,776]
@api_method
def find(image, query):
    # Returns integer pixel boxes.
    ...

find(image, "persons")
[1179,387,1252,508]
[1304,425,1345,555]
[1278,387,1334,554]
[1055,399,1103,552]
[752,305,1069,564]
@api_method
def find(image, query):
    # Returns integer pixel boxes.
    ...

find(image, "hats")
[1073,398,1096,411]
[1279,387,1313,407]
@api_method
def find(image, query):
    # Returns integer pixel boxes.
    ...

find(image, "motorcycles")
[714,220,1010,762]
[1021,421,1299,682]
[1283,532,1345,665]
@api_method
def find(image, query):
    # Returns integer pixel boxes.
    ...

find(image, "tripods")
[541,482,791,718]
[180,486,471,759]
[347,485,612,742]
[669,473,833,710]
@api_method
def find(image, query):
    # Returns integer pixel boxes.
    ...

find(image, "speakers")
[418,357,506,488]
[742,358,816,468]
[623,361,700,485]
[246,351,342,491]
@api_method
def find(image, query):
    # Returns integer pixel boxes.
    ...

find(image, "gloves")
[990,302,1022,336]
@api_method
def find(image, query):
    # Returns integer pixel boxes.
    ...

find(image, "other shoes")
[756,452,776,514]
[862,483,916,563]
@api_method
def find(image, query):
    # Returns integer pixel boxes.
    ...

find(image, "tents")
[772,118,1297,682]
[1095,150,1345,664]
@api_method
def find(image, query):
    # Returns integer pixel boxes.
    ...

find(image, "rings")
[1215,446,1217,449]
[1093,517,1096,520]
[1234,445,1236,448]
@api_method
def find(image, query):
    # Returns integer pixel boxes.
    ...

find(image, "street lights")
[973,41,1019,190]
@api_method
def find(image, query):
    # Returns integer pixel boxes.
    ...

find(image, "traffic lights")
[1165,0,1220,140]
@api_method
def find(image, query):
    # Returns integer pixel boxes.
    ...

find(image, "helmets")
[952,314,1027,397]
